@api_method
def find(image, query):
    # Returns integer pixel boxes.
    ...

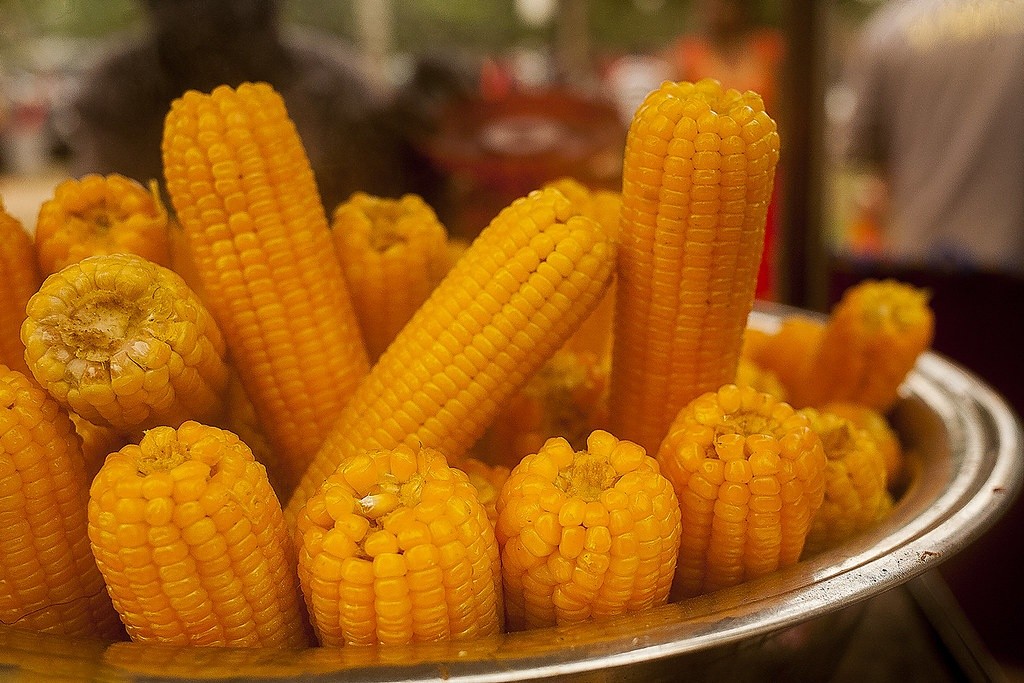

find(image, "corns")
[0,79,931,653]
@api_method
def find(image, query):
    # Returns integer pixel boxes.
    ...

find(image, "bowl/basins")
[1,302,1023,683]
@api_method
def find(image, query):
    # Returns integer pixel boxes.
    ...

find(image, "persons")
[0,0,1024,682]
